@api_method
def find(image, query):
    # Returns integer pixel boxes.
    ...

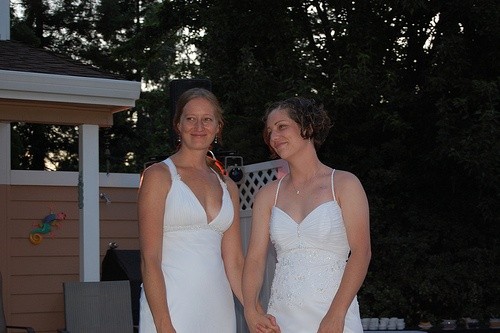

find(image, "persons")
[243,96,372,333]
[138,88,280,333]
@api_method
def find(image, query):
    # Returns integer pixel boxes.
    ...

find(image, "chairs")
[57,280,139,333]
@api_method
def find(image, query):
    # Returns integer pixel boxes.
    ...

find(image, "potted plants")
[416,277,500,333]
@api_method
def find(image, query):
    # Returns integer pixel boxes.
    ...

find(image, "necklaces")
[295,187,302,195]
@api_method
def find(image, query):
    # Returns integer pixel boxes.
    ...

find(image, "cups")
[442,320,455,330]
[466,319,479,329]
[490,319,500,328]
[362,318,404,330]
